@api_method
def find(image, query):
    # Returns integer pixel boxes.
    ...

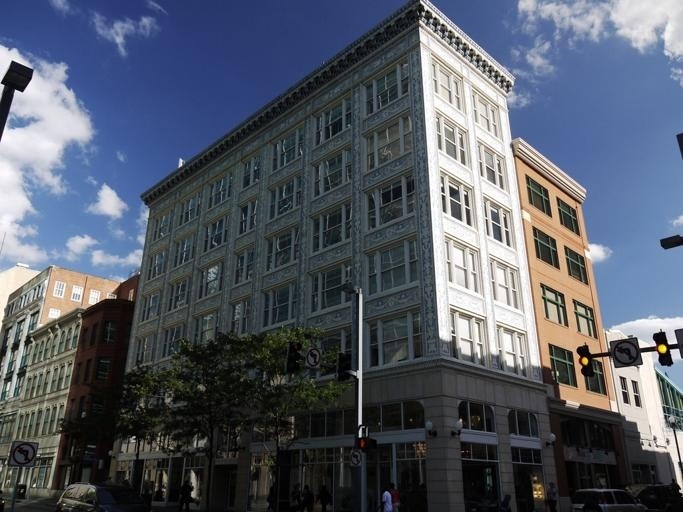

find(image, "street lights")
[338,280,364,436]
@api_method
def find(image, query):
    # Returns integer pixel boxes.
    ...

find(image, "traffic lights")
[576,345,596,378]
[652,330,674,366]
[353,434,378,462]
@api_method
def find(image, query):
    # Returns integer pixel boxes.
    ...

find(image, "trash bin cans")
[16,484,26,499]
[560,497,572,512]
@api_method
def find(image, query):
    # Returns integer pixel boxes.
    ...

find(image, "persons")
[178,480,194,511]
[381,485,392,511]
[499,494,512,511]
[299,483,314,512]
[314,484,330,511]
[266,487,276,512]
[546,481,558,511]
[386,483,400,512]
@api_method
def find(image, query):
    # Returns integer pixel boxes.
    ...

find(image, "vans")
[568,484,682,512]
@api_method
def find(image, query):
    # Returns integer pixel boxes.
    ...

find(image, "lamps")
[450,419,462,437]
[545,433,557,448]
[424,416,437,437]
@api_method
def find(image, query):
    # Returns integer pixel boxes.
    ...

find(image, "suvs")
[54,482,151,511]
